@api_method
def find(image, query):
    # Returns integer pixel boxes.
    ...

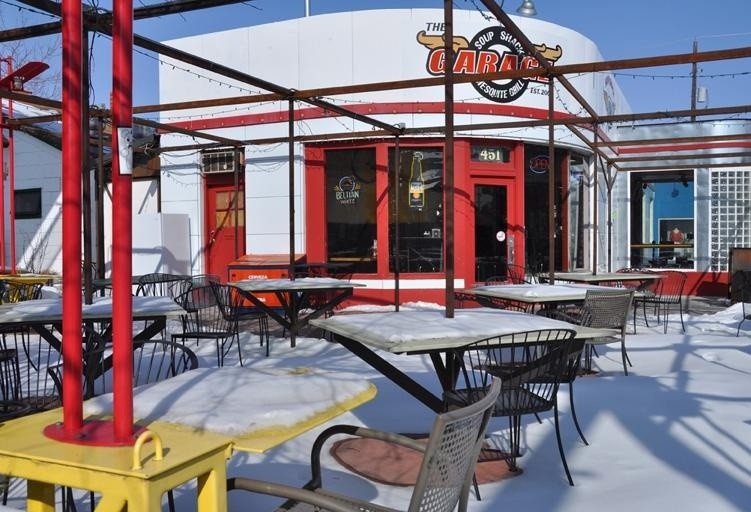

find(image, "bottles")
[408,156,425,208]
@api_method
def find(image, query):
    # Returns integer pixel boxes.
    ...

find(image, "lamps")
[516,0,538,16]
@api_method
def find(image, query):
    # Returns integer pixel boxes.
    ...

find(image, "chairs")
[729,247,751,337]
